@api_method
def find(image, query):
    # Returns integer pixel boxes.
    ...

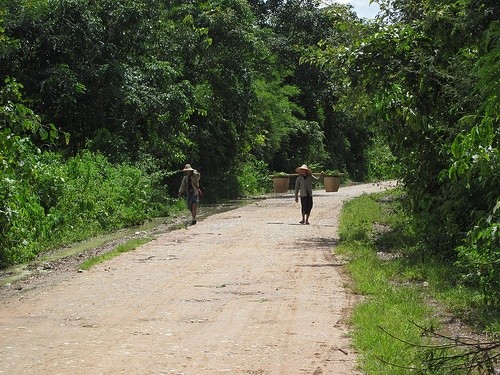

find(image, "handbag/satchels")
[198,188,202,197]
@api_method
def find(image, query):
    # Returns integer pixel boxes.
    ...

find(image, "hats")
[295,164,312,174]
[181,164,195,172]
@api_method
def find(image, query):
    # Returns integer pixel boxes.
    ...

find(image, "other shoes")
[191,220,196,225]
[299,221,306,223]
[305,221,310,224]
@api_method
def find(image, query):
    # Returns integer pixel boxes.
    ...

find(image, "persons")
[295,164,324,225]
[178,164,200,225]
